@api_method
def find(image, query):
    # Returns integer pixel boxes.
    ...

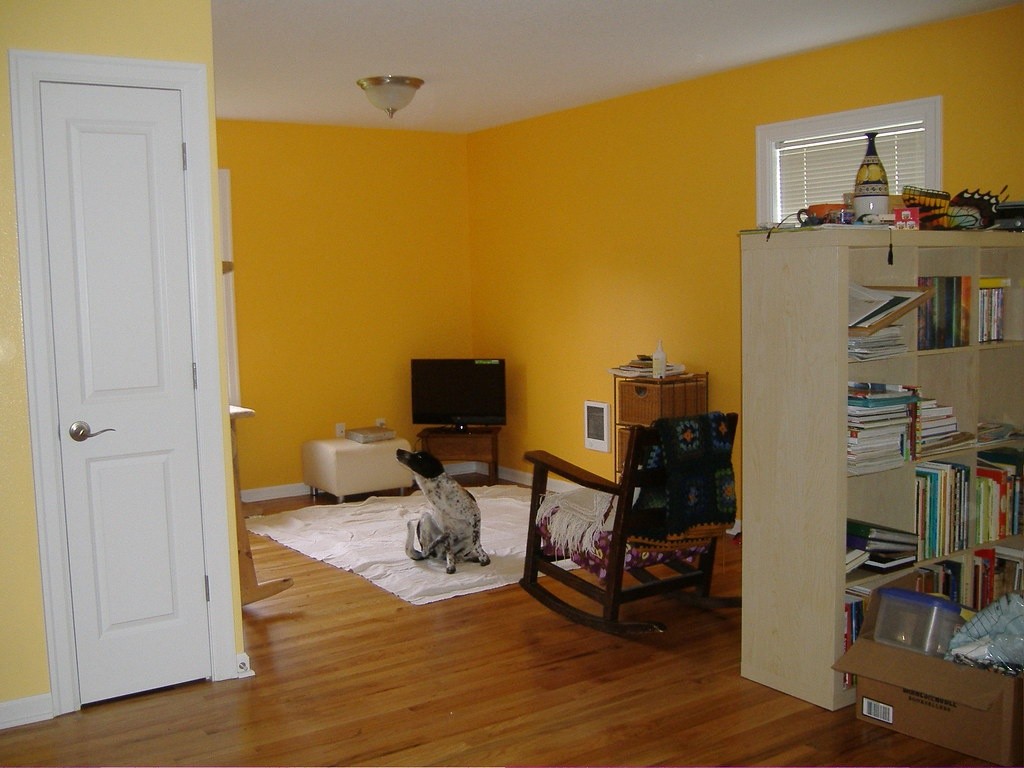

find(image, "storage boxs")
[831,571,1024,768]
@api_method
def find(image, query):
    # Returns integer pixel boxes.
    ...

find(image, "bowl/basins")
[797,204,847,226]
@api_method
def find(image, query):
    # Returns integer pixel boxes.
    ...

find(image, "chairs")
[518,411,741,635]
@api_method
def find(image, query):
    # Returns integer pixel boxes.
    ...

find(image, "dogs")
[396,448,492,575]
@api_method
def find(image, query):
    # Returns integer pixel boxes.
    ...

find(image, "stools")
[302,435,413,503]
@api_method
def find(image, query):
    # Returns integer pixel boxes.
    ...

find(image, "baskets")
[617,381,707,426]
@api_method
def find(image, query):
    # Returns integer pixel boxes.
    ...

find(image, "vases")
[852,133,889,226]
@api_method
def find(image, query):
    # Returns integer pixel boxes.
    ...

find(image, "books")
[977,451,1024,544]
[847,324,909,359]
[844,570,913,686]
[978,275,1011,343]
[846,550,918,575]
[915,460,971,561]
[738,223,888,236]
[915,546,1024,611]
[917,275,973,350]
[848,381,1024,475]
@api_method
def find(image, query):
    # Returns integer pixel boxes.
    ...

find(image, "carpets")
[243,485,582,603]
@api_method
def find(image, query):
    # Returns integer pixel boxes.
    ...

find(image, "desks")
[417,426,502,486]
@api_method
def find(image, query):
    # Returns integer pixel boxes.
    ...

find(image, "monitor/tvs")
[411,358,506,433]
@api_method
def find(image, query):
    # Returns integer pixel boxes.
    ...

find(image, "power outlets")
[336,423,346,437]
[376,418,385,427]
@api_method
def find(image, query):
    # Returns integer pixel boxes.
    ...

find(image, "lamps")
[357,75,425,119]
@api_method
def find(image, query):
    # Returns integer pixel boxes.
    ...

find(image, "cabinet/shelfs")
[740,228,1024,712]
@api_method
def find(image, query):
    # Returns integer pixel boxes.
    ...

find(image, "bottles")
[854,132,889,223]
[652,340,667,378]
